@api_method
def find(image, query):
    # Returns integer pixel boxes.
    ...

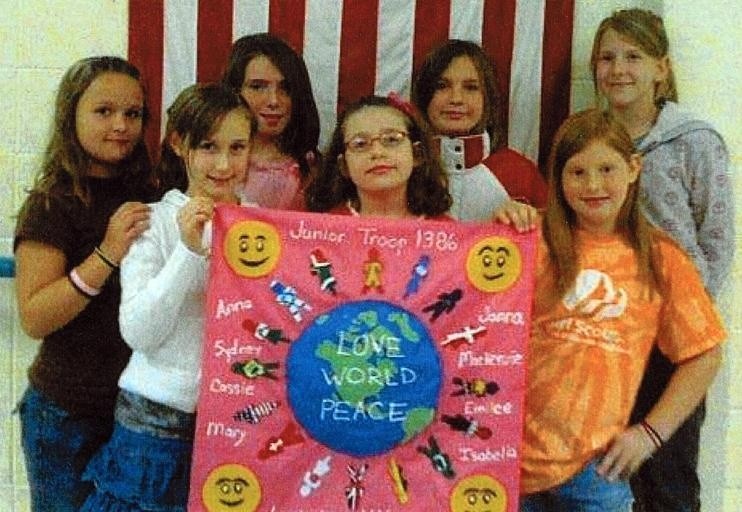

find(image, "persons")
[15,54,165,511]
[305,95,459,222]
[590,8,732,512]
[488,109,729,512]
[221,33,320,212]
[415,36,551,223]
[76,80,257,511]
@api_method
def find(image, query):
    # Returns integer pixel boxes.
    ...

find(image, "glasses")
[342,129,412,153]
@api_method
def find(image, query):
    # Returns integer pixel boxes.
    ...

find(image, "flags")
[126,0,573,189]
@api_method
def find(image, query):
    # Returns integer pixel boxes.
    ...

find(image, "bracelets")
[68,268,102,299]
[94,247,119,269]
[639,419,665,451]
